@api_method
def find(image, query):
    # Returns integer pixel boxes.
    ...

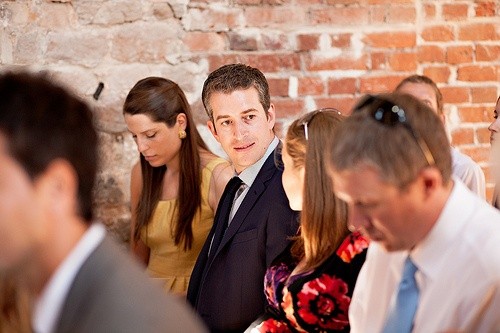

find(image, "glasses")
[355,95,436,166]
[297,107,341,143]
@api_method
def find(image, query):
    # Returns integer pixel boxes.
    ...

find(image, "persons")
[489,94,500,211]
[394,73,487,201]
[246,105,371,333]
[0,65,211,333]
[324,90,499,332]
[123,76,236,306]
[184,62,300,332]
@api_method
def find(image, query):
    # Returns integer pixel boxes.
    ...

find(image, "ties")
[205,177,243,270]
[383,256,418,333]
